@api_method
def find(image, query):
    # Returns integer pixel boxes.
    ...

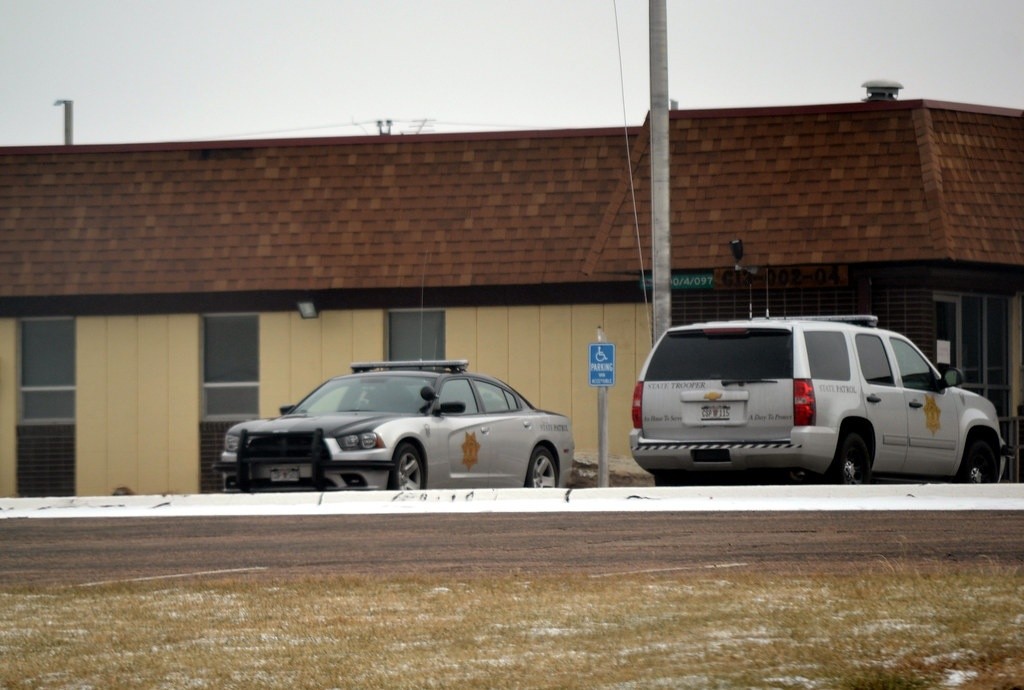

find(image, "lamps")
[730,240,744,265]
[296,296,320,319]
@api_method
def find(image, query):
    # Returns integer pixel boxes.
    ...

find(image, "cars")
[211,363,576,495]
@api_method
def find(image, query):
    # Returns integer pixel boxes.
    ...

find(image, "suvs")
[628,313,1013,491]
